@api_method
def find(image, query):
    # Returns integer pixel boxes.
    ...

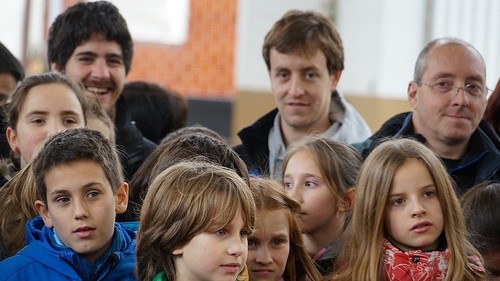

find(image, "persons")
[231,10,372,190]
[0,37,500,281]
[46,0,160,185]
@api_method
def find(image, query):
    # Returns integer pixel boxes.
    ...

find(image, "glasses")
[419,80,494,99]
